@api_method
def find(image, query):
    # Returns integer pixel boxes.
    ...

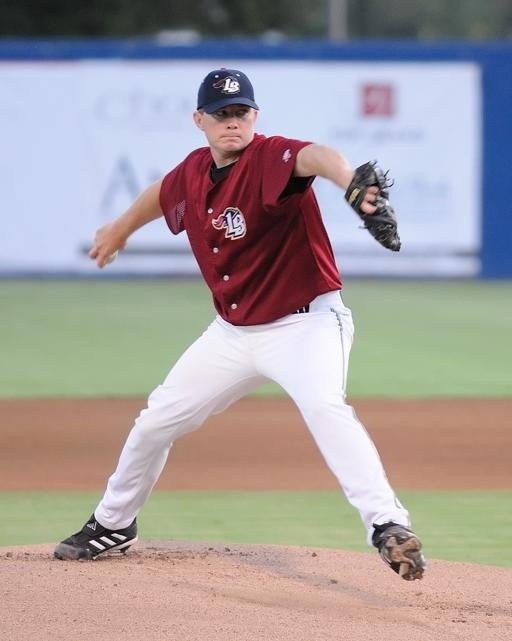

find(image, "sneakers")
[54,511,138,561]
[372,522,425,581]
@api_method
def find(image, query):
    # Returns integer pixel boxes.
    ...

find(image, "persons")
[54,70,426,581]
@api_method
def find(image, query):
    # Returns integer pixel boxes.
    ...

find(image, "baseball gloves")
[345,162,402,252]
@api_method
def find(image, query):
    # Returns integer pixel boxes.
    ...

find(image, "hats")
[197,67,260,114]
